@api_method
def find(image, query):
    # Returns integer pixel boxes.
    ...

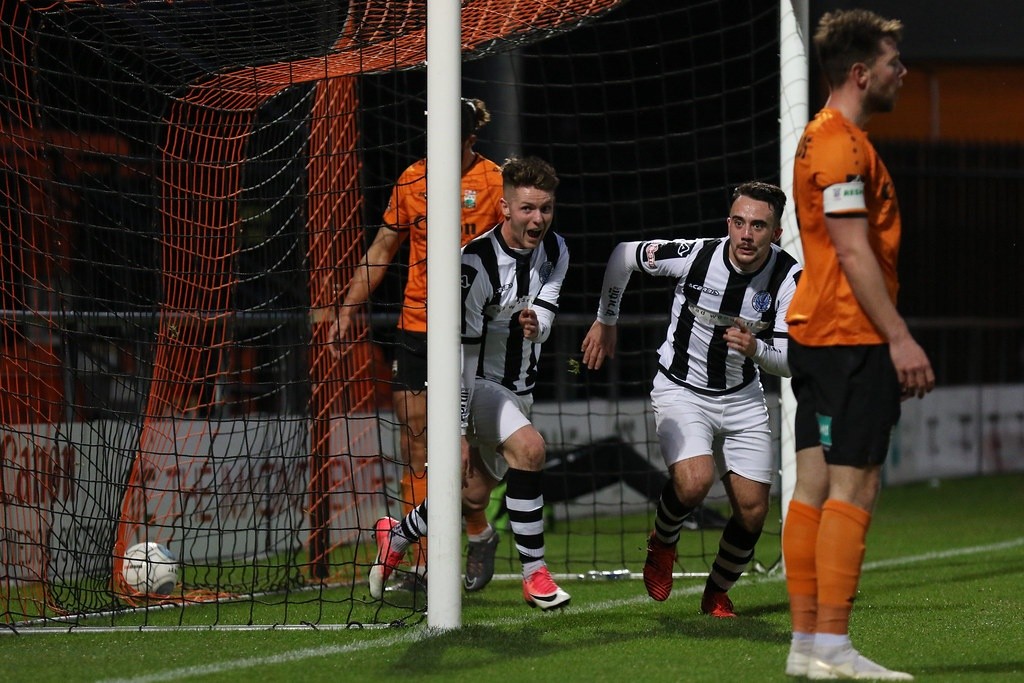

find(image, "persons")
[0,290,394,423]
[785,10,934,683]
[368,158,572,612]
[580,181,802,618]
[328,96,506,593]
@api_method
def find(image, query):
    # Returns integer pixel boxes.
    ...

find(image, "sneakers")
[785,638,811,677]
[643,530,680,601]
[701,590,738,618]
[807,642,914,681]
[464,523,499,591]
[522,566,571,610]
[368,516,405,599]
[396,568,429,591]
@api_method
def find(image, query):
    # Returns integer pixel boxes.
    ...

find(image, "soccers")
[122,541,178,603]
